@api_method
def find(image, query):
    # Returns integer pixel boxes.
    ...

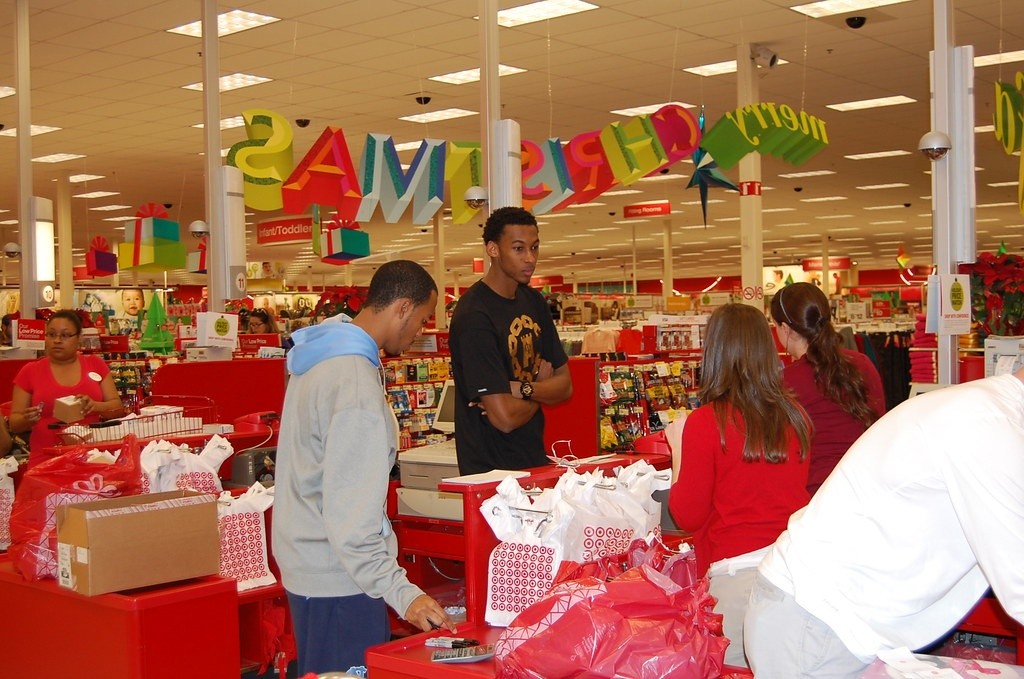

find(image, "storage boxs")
[56,490,221,598]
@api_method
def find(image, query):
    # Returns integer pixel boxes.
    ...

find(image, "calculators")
[431,643,495,663]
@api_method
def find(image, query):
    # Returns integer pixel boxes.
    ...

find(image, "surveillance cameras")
[307,223,484,277]
[660,168,671,174]
[904,203,911,207]
[188,220,209,240]
[164,204,172,208]
[919,130,953,162]
[572,251,625,270]
[4,242,20,258]
[793,188,802,192]
[416,97,430,105]
[296,119,309,127]
[753,48,778,70]
[463,187,488,209]
[609,212,615,215]
[0,124,4,131]
[845,17,867,29]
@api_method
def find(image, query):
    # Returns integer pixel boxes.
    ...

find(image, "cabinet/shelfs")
[0,281,1024,679]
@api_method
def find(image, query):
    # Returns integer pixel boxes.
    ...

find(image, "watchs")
[519,380,533,399]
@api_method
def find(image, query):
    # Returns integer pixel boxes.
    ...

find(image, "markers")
[424,636,479,649]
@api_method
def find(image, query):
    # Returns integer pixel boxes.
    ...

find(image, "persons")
[122,290,145,316]
[833,272,840,294]
[270,260,457,679]
[450,207,573,476]
[770,281,886,495]
[665,304,812,669]
[812,275,822,287]
[84,293,103,309]
[886,306,914,411]
[0,310,123,471]
[774,270,785,291]
[246,308,309,357]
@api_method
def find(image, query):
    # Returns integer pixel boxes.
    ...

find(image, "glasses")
[44,331,78,340]
[250,322,263,328]
[253,308,267,316]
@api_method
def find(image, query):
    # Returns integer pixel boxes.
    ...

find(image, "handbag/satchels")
[9,434,144,584]
[492,537,731,679]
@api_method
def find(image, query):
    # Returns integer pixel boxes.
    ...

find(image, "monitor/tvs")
[431,379,457,434]
